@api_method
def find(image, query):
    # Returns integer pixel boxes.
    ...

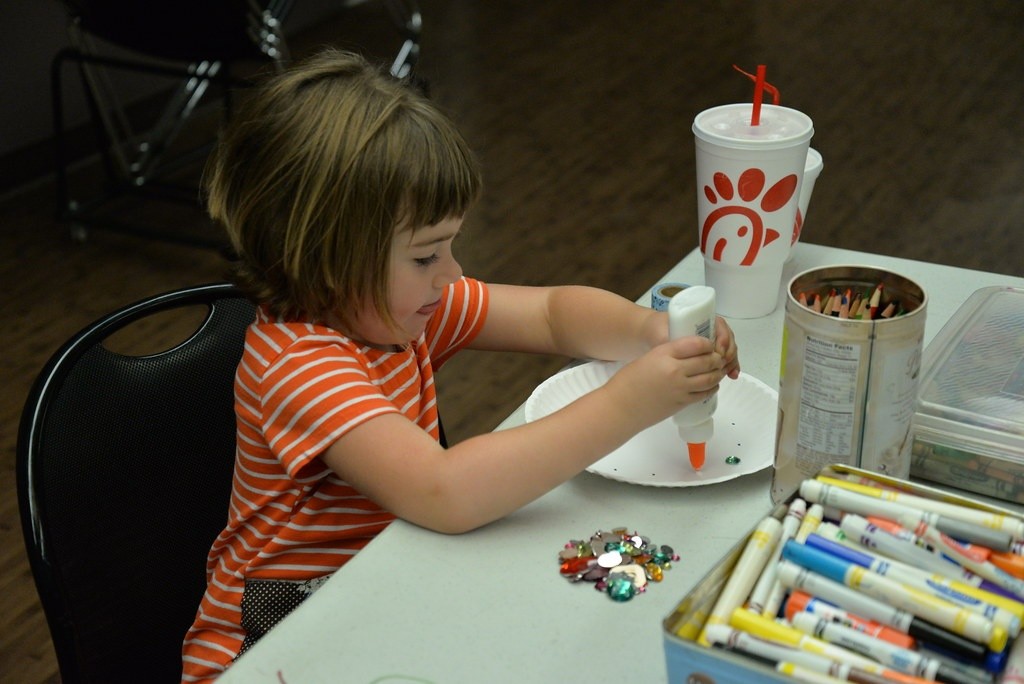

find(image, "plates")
[524,361,779,488]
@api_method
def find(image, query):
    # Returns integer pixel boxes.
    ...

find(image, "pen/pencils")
[799,284,905,320]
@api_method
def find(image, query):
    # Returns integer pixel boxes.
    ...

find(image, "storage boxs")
[900,284,1024,505]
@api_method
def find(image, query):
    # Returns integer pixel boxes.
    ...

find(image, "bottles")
[668,285,717,445]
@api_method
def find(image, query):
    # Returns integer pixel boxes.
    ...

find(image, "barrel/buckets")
[770,262,931,501]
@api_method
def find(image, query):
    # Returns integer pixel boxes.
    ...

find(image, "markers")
[697,469,1024,684]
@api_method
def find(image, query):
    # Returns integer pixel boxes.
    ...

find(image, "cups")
[692,102,815,318]
[782,147,825,263]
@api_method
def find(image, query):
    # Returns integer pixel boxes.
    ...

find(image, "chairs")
[16,281,446,684]
[46,0,427,264]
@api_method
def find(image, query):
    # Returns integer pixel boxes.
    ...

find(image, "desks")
[204,237,1024,684]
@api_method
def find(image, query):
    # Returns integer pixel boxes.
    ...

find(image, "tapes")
[650,282,692,310]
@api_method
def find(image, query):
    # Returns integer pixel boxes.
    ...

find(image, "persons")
[181,54,740,684]
